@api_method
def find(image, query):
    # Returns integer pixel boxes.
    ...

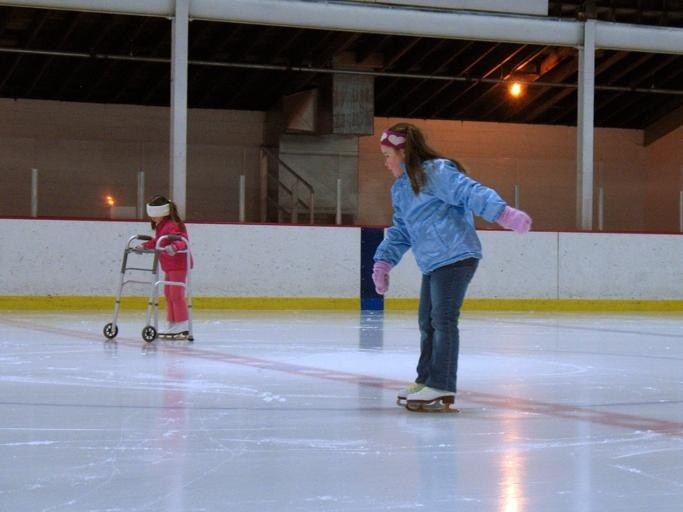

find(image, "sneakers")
[398,383,455,403]
[157,320,189,335]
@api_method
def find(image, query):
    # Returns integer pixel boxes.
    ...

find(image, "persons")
[369,122,533,405]
[131,193,194,335]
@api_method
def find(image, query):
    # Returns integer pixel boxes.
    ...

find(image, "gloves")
[496,206,532,233]
[165,245,175,257]
[133,244,144,254]
[372,261,391,295]
